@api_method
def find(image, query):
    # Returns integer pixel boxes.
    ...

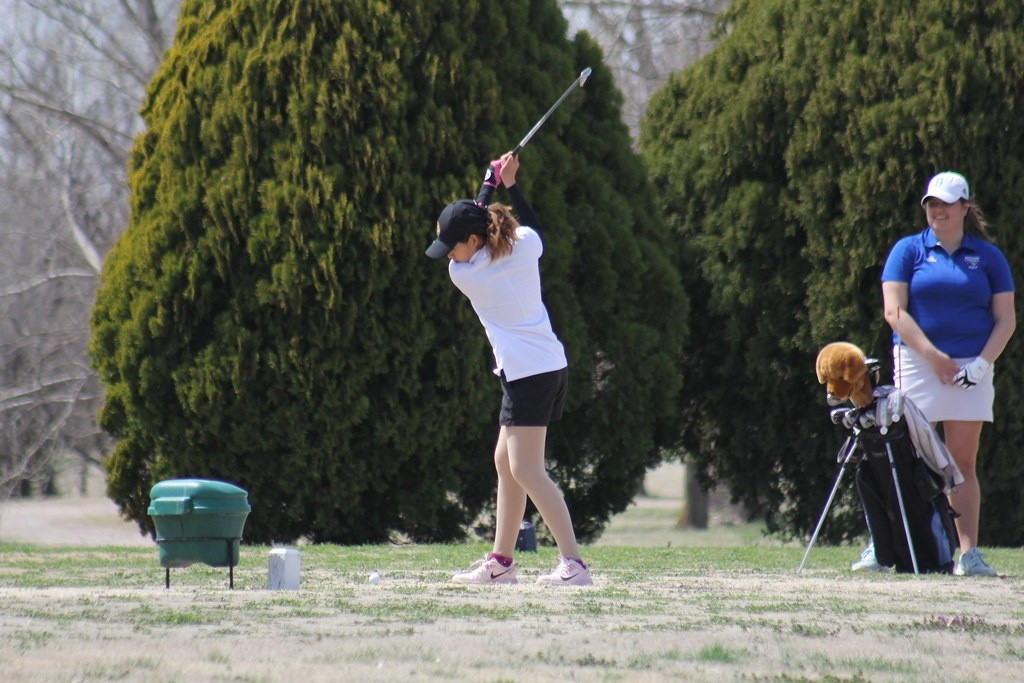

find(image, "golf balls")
[368,572,380,585]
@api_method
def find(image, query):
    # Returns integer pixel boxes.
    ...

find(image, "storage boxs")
[148,480,249,567]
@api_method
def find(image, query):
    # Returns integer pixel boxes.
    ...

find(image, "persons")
[423,149,597,587]
[851,170,1018,576]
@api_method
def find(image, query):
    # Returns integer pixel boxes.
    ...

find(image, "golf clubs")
[824,358,906,436]
[507,66,592,161]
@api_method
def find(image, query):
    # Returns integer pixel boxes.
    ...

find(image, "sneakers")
[851,542,893,573]
[955,547,997,576]
[537,559,594,586]
[451,557,518,584]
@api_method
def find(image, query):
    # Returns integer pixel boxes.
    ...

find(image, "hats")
[921,171,969,206]
[425,199,493,260]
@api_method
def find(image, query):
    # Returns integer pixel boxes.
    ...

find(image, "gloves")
[476,159,501,207]
[952,356,990,391]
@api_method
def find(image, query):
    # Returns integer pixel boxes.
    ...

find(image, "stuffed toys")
[814,342,876,411]
